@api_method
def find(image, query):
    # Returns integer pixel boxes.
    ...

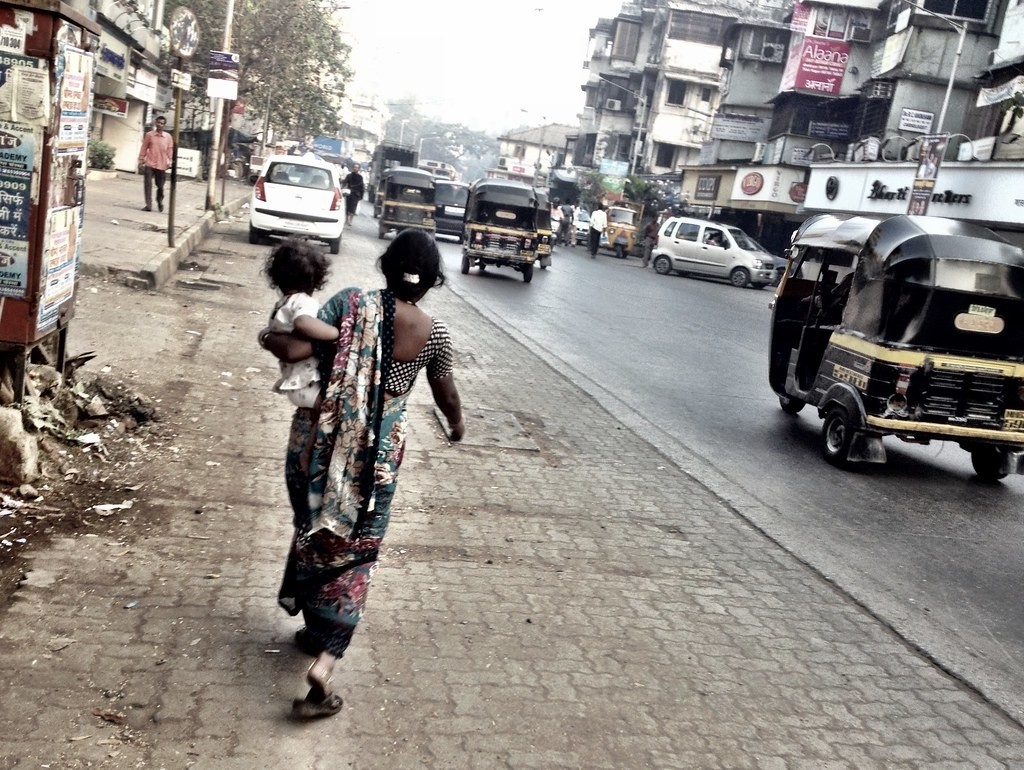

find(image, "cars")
[248,155,350,253]
[552,206,590,244]
[436,180,469,243]
[732,234,789,281]
[649,215,777,288]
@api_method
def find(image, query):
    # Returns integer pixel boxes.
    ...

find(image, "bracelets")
[261,332,270,344]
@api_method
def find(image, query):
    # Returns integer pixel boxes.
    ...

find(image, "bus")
[420,161,458,180]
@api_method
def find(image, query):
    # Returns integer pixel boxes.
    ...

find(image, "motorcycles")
[374,166,436,240]
[534,191,553,270]
[769,210,1024,479]
[604,207,639,259]
[460,178,539,282]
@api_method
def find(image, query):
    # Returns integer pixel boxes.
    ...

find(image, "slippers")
[292,693,341,719]
[296,626,320,658]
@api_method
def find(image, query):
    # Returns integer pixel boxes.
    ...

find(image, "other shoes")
[142,207,151,211]
[157,199,164,212]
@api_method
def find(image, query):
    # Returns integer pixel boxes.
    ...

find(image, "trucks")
[368,140,418,202]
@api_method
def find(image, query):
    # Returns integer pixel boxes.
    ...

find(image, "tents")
[168,125,258,151]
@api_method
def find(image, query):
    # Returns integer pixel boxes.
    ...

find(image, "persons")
[138,116,173,212]
[642,216,660,267]
[589,202,608,256]
[708,233,721,246]
[917,142,937,179]
[279,229,464,718]
[259,233,339,478]
[801,246,903,342]
[550,197,564,233]
[339,162,364,227]
[559,198,574,246]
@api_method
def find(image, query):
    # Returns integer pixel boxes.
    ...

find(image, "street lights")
[520,109,546,189]
[592,73,645,208]
[413,132,440,159]
[399,119,410,148]
